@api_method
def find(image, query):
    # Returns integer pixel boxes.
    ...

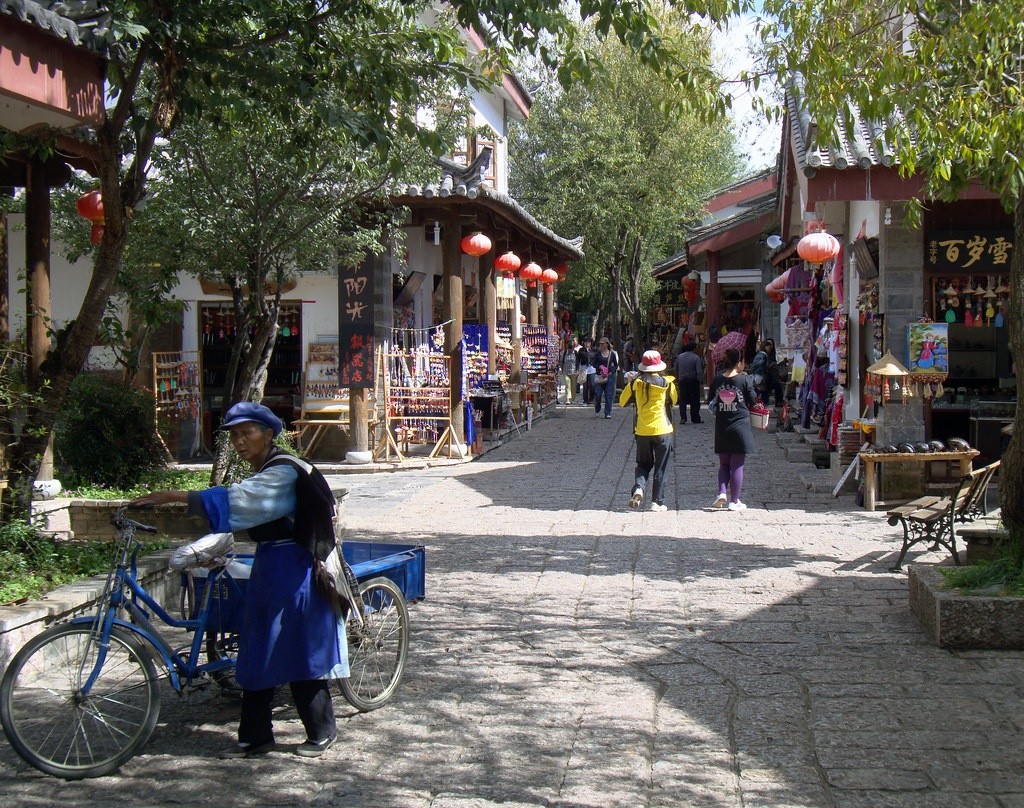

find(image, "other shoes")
[693,420,705,423]
[565,400,570,404]
[583,403,587,407]
[296,732,338,757]
[680,416,687,424]
[596,409,601,416]
[216,735,275,759]
[605,415,611,418]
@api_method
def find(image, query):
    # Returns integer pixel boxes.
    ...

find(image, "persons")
[715,338,783,407]
[619,350,678,511]
[127,402,351,758]
[559,336,705,424]
[707,349,759,510]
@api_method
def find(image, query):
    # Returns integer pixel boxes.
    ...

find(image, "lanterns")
[681,276,698,306]
[462,231,492,257]
[539,255,569,293]
[495,250,521,281]
[764,270,792,304]
[520,314,526,323]
[796,228,841,271]
[519,261,542,287]
[77,186,105,244]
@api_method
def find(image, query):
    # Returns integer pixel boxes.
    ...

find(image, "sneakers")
[652,502,667,512]
[713,493,727,508]
[729,499,747,511]
[628,488,643,508]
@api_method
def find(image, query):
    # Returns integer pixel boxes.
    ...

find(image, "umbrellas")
[710,331,748,363]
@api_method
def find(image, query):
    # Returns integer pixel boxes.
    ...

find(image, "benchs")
[887,459,1001,571]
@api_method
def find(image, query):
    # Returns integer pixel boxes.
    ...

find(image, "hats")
[218,402,283,437]
[597,338,609,345]
[866,354,908,376]
[638,350,666,372]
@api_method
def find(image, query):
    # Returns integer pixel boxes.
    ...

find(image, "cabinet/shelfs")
[937,321,998,386]
[969,398,1017,468]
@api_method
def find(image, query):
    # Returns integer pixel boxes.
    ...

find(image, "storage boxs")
[509,389,522,410]
[513,408,522,425]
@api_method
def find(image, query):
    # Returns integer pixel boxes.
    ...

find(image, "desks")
[291,417,379,461]
[858,447,981,511]
[468,391,510,448]
[527,392,539,416]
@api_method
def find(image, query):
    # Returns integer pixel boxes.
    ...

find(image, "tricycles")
[0,503,424,778]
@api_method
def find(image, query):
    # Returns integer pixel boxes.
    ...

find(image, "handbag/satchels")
[594,374,608,384]
[750,401,769,430]
[577,369,588,385]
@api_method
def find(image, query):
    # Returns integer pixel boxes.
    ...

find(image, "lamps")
[688,267,701,280]
